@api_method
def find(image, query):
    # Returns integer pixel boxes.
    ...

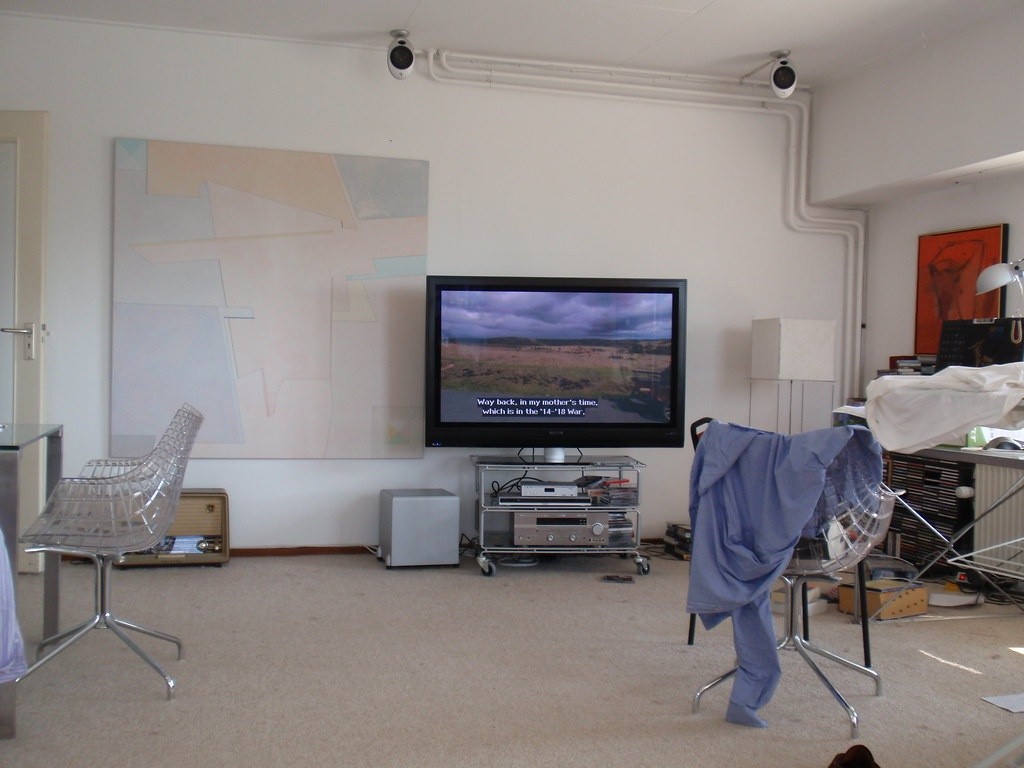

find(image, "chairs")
[19,403,204,701]
[691,417,907,739]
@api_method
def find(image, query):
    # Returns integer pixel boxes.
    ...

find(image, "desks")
[867,445,1024,624]
[0,424,63,644]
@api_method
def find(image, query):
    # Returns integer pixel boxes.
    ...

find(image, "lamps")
[748,316,838,436]
[975,259,1024,298]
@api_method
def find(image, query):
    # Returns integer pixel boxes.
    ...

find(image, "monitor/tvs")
[426,274,687,465]
[935,318,1024,372]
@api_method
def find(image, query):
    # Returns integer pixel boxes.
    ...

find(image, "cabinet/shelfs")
[113,489,230,571]
[474,455,650,577]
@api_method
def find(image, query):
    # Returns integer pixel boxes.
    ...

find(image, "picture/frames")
[914,223,1009,357]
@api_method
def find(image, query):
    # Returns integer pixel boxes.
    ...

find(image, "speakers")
[769,49,797,99]
[387,30,415,79]
[114,488,230,565]
[379,487,460,567]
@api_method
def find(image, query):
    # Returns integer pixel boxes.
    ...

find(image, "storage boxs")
[839,579,928,621]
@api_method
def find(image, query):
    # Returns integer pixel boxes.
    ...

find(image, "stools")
[376,488,460,570]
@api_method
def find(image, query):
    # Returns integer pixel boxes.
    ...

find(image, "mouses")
[981,437,1021,451]
